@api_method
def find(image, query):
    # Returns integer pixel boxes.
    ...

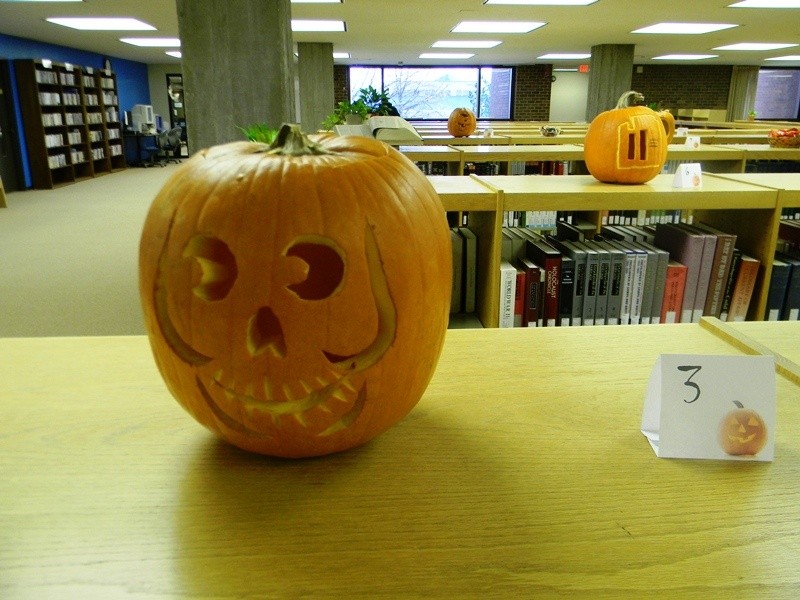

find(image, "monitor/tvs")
[136,105,154,124]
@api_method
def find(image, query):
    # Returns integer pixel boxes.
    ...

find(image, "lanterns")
[448,107,474,137]
[652,107,675,146]
[583,90,670,185]
[137,124,450,461]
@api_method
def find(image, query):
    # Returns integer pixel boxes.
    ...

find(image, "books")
[28,65,123,170]
[396,134,800,330]
[331,113,424,144]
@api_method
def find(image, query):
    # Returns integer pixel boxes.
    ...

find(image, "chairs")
[145,130,172,169]
[159,126,186,166]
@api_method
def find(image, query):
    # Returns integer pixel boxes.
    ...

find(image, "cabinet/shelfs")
[13,55,130,190]
[0,103,799,600]
